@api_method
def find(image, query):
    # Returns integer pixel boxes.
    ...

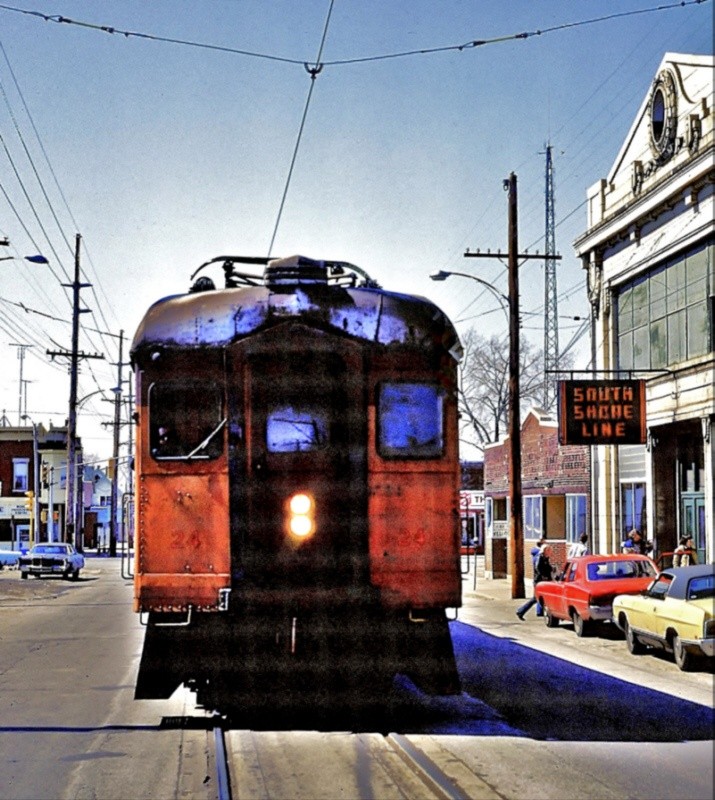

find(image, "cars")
[534,554,672,638]
[0,550,22,571]
[18,543,85,579]
[610,564,715,673]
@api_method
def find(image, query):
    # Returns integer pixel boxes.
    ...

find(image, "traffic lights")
[24,491,34,510]
[40,465,49,483]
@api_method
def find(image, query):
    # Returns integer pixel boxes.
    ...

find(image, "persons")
[566,531,588,572]
[516,542,553,619]
[672,533,700,568]
[623,529,653,573]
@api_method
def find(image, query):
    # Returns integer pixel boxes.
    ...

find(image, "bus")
[130,255,472,713]
[459,506,485,554]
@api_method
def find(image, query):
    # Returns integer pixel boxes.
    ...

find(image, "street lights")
[426,267,527,600]
[63,386,125,542]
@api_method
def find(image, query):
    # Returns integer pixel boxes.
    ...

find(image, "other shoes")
[516,610,524,621]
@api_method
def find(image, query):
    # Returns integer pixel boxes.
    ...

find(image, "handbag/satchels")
[561,560,568,571]
